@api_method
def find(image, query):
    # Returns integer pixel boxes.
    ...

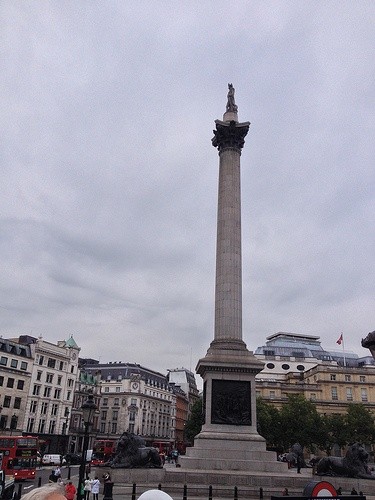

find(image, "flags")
[336,335,343,345]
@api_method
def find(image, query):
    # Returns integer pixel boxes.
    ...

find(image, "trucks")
[0,471,15,497]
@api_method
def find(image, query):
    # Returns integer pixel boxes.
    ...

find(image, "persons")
[80,476,100,500]
[226,84,235,111]
[336,487,342,495]
[21,483,67,500]
[65,481,76,500]
[351,487,357,495]
[49,466,62,483]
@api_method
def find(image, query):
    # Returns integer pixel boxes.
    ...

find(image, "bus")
[0,435,38,481]
[90,440,118,466]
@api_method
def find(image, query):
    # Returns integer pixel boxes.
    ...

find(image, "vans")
[43,455,66,466]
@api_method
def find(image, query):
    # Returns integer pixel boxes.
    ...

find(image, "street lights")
[61,411,70,454]
[77,393,98,500]
